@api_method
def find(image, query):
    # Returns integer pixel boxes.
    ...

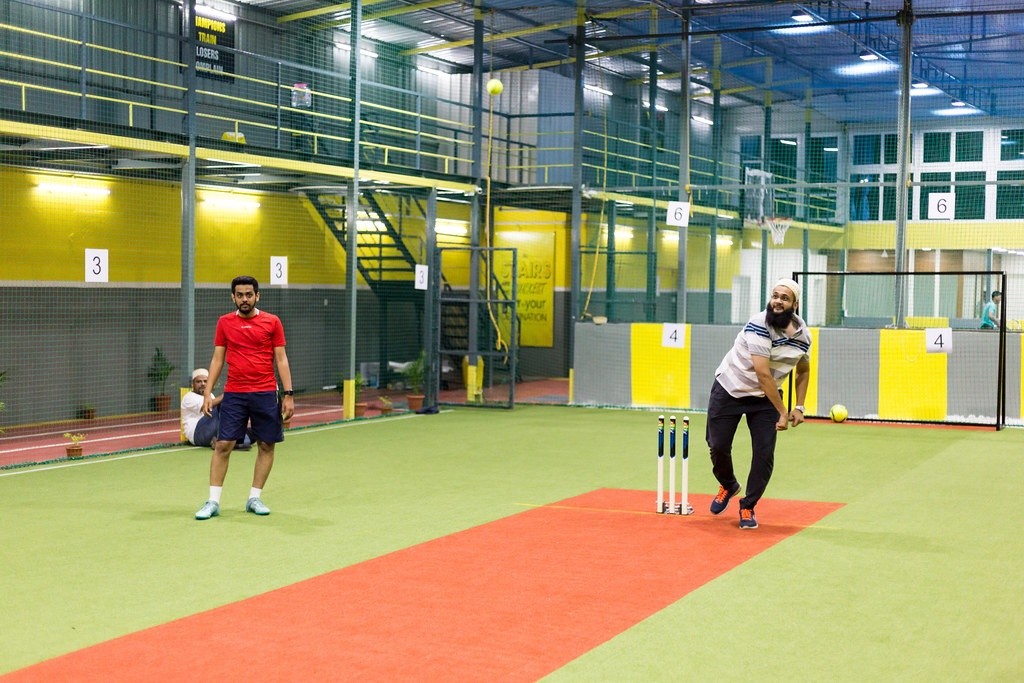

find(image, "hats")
[992,291,1002,297]
[772,277,801,302]
[191,368,209,379]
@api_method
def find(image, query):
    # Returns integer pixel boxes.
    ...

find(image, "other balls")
[830,404,848,422]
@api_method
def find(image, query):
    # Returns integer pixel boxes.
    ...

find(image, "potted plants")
[378,395,392,417]
[393,351,426,411]
[63,432,87,457]
[147,347,175,413]
[336,367,367,418]
[75,403,96,419]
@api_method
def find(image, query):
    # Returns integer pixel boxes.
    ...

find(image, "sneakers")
[711,483,742,515]
[739,506,759,530]
[246,498,269,515]
[194,500,220,519]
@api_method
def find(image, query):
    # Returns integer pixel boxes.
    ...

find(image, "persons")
[979,291,1002,329]
[194,276,294,520]
[706,279,812,530]
[181,368,256,450]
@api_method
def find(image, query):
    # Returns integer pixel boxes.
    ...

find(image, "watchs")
[795,406,805,413]
[283,390,293,396]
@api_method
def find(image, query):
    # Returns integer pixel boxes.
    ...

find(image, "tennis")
[486,79,503,95]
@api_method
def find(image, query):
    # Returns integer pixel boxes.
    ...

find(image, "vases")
[284,421,290,430]
